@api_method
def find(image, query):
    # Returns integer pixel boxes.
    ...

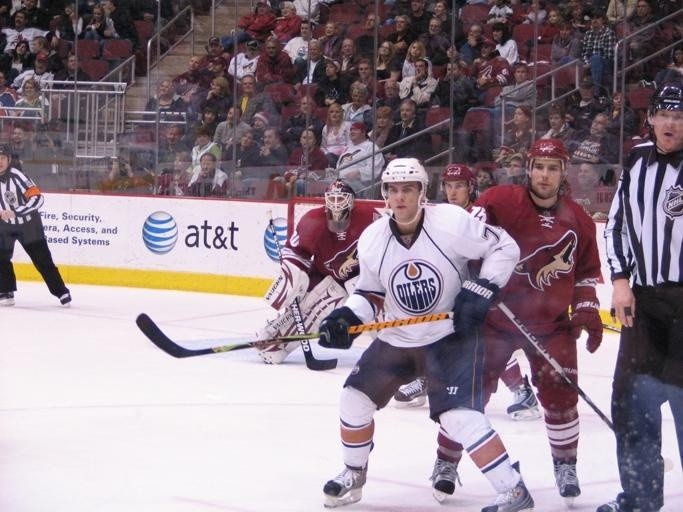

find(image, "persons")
[0,143,71,307]
[286,178,381,295]
[1,0,683,223]
[428,138,604,497]
[315,157,535,512]
[596,76,683,512]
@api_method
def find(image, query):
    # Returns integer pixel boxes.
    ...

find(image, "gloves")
[572,285,603,352]
[453,279,498,330]
[318,307,362,348]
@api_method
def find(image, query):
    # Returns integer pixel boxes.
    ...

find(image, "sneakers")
[60,290,71,304]
[324,465,367,496]
[0,291,13,299]
[553,459,629,511]
[431,458,457,494]
[395,379,425,400]
[507,387,537,413]
[481,481,534,512]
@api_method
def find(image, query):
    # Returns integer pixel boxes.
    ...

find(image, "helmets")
[528,139,566,180]
[443,163,477,199]
[380,157,428,207]
[324,180,355,221]
[653,80,683,111]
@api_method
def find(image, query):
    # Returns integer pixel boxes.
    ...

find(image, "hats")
[37,53,49,63]
[482,39,496,48]
[255,111,270,125]
[351,123,366,132]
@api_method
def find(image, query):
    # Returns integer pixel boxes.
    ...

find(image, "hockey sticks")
[134,313,458,357]
[266,210,338,370]
